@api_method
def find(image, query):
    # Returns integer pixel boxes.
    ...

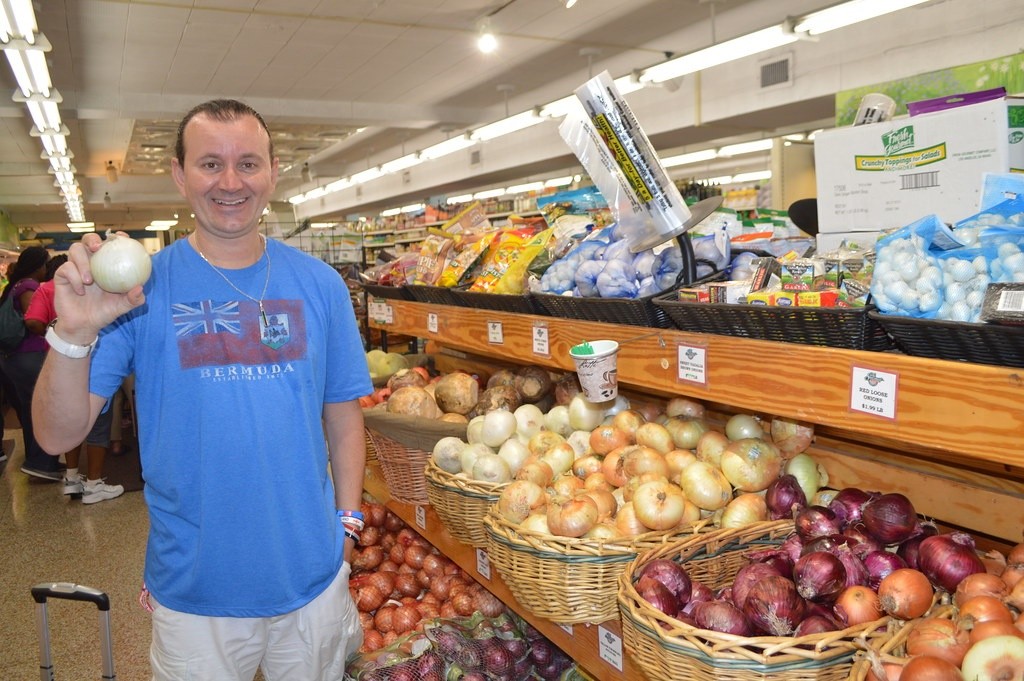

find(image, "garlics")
[529,223,760,301]
[871,211,1024,323]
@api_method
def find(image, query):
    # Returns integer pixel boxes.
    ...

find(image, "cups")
[568,340,618,403]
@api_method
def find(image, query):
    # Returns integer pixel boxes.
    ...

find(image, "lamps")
[0,1,941,245]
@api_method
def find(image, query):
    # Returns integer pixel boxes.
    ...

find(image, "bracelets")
[44,318,98,358]
[337,509,365,543]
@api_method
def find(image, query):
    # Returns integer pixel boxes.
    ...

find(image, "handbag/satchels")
[0,285,27,353]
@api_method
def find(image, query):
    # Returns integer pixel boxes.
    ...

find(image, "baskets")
[363,251,1024,368]
[361,399,961,681]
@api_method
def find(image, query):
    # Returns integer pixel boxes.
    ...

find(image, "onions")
[89,228,151,294]
[348,391,1024,681]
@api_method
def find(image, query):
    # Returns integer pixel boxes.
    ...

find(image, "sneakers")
[63,473,88,494]
[20,458,66,480]
[82,480,123,504]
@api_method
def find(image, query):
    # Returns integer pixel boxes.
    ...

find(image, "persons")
[0,246,127,503]
[32,100,374,681]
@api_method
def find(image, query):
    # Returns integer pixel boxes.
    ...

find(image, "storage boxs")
[679,95,1024,310]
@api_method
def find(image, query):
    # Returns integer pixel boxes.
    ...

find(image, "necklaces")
[194,230,271,326]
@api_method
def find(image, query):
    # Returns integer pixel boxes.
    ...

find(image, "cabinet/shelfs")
[360,294,1024,681]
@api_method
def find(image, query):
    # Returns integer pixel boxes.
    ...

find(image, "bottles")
[348,176,591,262]
[673,175,721,202]
